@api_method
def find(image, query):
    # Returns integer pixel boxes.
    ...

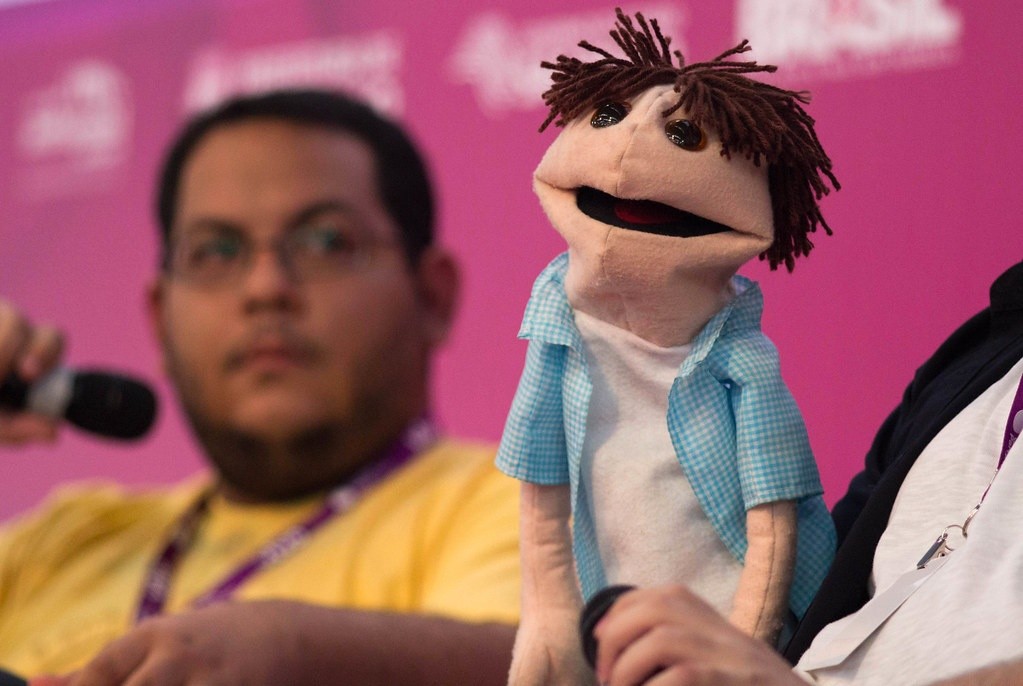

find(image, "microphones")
[1,371,157,441]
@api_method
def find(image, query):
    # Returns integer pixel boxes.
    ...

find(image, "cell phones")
[579,586,665,686]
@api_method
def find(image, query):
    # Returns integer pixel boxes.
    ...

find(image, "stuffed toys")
[495,10,838,685]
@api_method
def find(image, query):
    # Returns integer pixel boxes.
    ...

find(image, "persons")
[597,256,1023,686]
[0,88,527,686]
